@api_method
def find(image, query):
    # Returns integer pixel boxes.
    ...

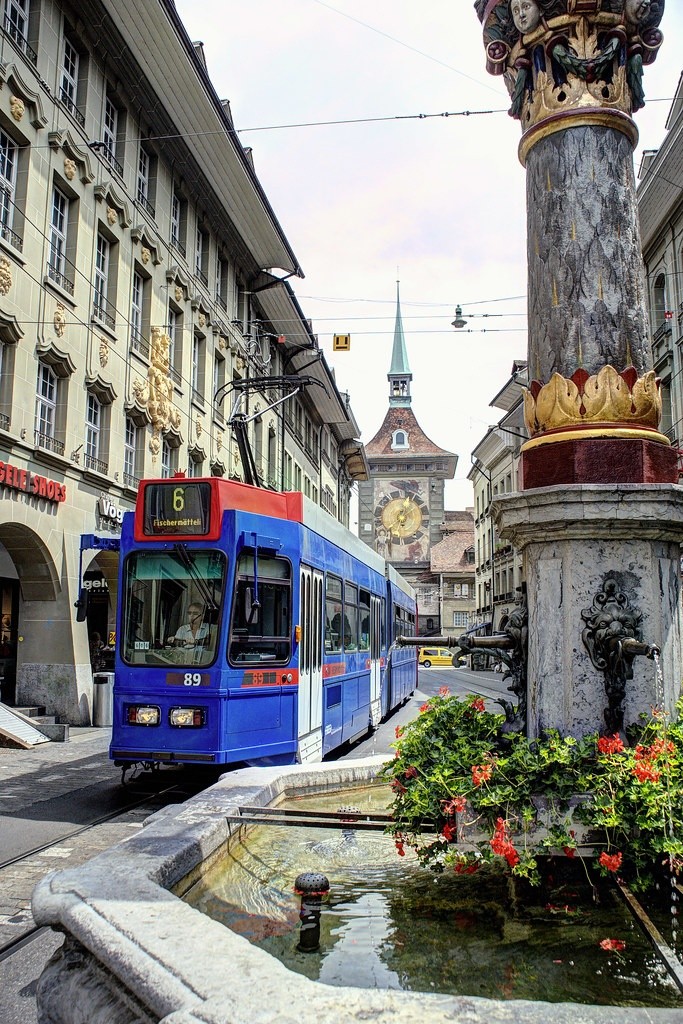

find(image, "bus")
[76,475,420,772]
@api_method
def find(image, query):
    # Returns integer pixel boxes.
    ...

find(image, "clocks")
[374,491,429,545]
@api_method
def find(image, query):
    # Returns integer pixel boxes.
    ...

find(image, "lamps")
[451,305,467,328]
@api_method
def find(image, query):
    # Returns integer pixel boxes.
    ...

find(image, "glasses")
[187,610,204,615]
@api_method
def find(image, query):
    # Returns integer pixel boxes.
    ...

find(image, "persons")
[91,632,104,648]
[167,602,214,647]
[338,630,367,650]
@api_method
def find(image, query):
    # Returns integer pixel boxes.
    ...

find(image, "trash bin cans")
[92,672,115,727]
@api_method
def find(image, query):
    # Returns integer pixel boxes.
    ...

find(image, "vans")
[419,647,467,668]
